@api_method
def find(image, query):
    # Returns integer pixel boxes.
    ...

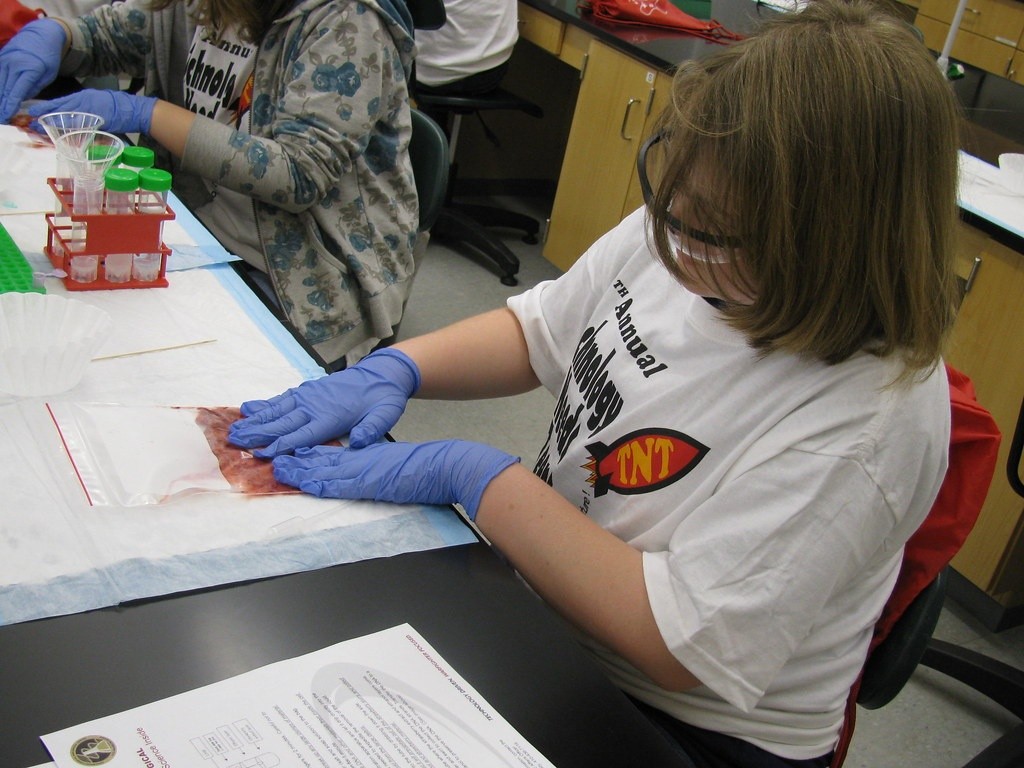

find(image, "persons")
[407,0,520,147]
[0,0,417,371]
[230,3,952,768]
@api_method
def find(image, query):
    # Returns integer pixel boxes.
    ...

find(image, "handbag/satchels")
[579,0,745,49]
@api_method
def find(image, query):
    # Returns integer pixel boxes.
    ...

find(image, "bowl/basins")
[0,292,113,395]
[998,153,1024,192]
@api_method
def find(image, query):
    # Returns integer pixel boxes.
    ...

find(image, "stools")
[413,88,544,285]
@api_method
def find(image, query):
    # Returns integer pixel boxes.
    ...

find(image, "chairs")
[372,109,449,351]
[832,364,1003,768]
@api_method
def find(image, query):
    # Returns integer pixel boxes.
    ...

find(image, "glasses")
[635,126,745,249]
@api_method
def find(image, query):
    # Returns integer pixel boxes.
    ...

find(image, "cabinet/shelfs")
[517,0,1024,635]
[913,0,1024,85]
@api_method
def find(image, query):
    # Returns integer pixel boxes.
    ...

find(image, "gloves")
[272,437,524,520]
[0,19,67,125]
[227,347,421,461]
[28,87,158,137]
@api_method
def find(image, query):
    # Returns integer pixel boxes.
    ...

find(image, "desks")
[0,76,692,768]
